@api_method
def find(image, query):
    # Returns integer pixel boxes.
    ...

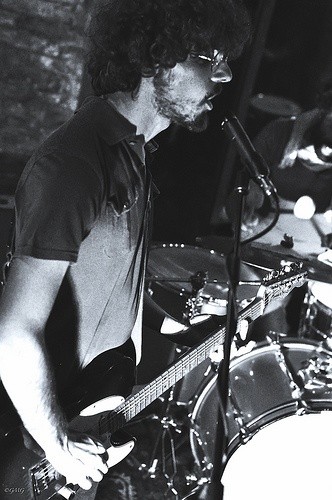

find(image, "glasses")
[198,47,231,72]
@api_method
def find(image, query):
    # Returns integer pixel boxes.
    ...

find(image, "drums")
[300,277,332,343]
[138,239,271,407]
[188,335,332,500]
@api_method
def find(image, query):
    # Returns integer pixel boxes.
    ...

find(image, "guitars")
[0,260,313,500]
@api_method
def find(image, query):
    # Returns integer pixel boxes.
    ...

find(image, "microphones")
[218,107,279,206]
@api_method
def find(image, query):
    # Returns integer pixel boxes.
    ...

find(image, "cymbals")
[227,239,331,280]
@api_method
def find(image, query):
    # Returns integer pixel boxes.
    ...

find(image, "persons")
[0,0,249,500]
